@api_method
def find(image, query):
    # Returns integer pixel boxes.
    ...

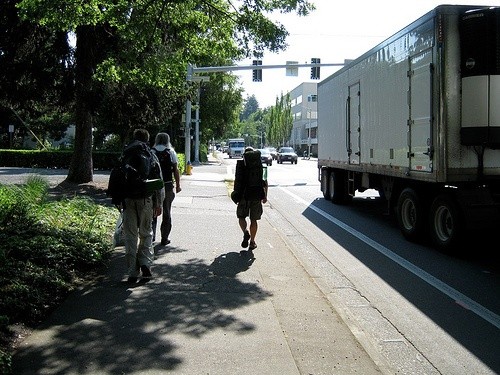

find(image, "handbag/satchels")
[113,209,125,246]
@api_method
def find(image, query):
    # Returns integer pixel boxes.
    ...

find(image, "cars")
[265,148,278,160]
[254,148,272,167]
[216,141,229,153]
[276,147,298,164]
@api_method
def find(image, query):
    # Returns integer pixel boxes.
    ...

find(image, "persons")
[150,133,181,245]
[237,147,268,249]
[107,130,164,288]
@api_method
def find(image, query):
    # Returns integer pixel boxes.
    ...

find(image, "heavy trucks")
[315,4,500,252]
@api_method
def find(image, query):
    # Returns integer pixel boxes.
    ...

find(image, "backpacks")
[231,150,267,205]
[120,143,163,199]
[151,147,176,174]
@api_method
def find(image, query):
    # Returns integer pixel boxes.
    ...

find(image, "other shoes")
[241,231,250,248]
[161,240,170,245]
[141,265,152,277]
[249,241,257,249]
[128,277,137,283]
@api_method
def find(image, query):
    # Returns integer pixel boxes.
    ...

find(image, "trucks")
[227,138,247,158]
